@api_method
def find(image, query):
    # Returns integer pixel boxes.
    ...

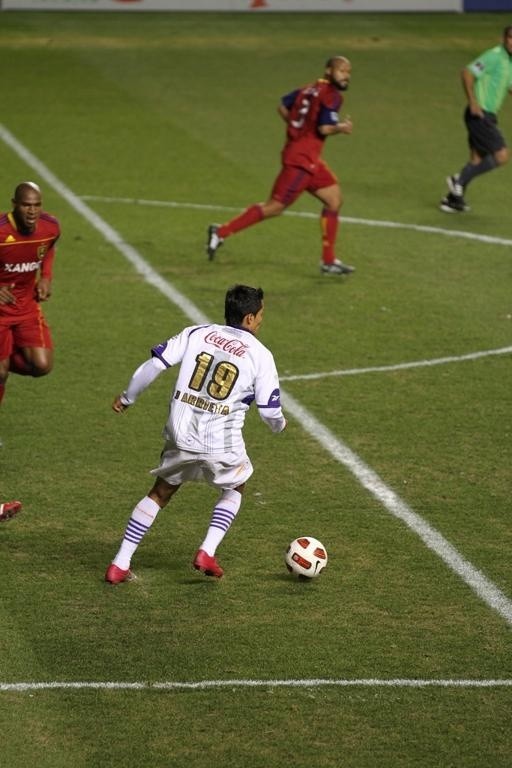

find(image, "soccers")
[285,537,328,581]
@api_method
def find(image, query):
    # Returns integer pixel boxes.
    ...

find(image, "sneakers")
[321,258,355,275]
[208,223,223,260]
[438,174,471,212]
[106,564,141,584]
[194,549,224,578]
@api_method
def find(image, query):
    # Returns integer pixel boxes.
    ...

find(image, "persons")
[0,182,62,403]
[207,56,356,276]
[105,285,287,583]
[440,26,512,214]
